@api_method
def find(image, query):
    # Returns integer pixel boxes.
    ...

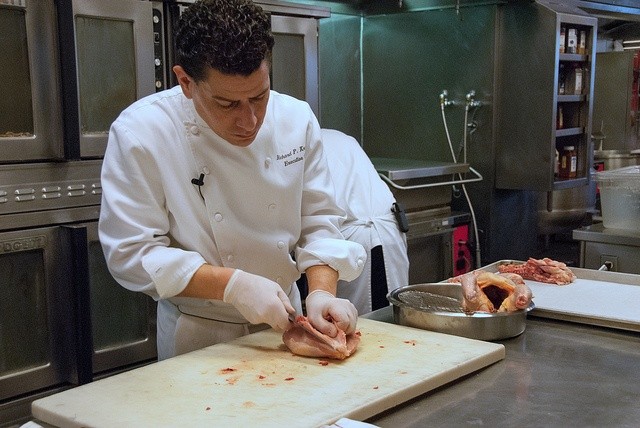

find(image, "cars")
[559,146,577,178]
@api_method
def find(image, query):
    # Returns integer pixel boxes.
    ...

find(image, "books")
[593,166,640,230]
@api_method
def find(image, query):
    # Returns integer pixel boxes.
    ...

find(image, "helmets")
[387,280,534,340]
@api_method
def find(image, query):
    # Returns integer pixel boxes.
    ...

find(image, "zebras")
[174,0,328,130]
[1,221,168,415]
[1,0,165,228]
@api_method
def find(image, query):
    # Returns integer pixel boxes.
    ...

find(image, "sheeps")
[463,90,480,112]
[436,88,451,111]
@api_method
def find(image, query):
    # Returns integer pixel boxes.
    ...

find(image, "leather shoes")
[223,268,296,333]
[305,290,358,338]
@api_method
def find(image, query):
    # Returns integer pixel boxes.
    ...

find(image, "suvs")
[594,149,640,213]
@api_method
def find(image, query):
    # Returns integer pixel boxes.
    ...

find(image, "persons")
[97,1,367,362]
[320,128,410,318]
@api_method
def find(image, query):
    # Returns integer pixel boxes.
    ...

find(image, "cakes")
[560,145,576,177]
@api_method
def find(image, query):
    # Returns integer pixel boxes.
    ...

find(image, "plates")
[494,0,598,192]
